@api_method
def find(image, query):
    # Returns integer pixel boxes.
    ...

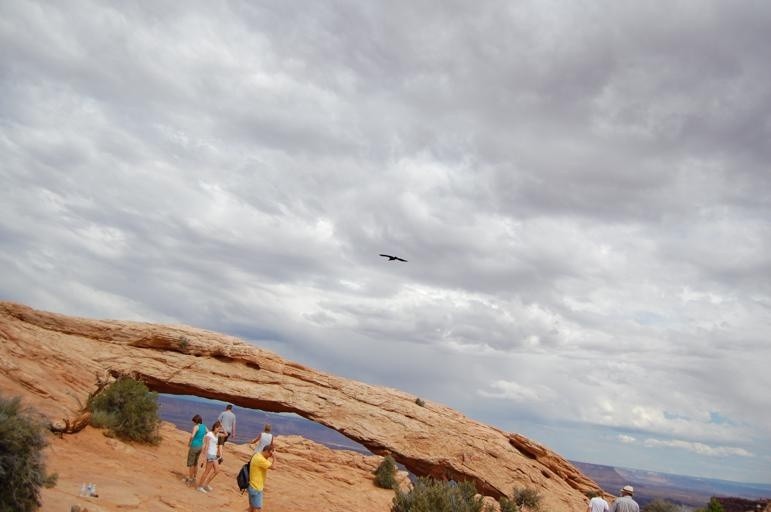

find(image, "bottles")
[79,481,96,496]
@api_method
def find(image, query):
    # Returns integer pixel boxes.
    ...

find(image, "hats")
[620,485,633,496]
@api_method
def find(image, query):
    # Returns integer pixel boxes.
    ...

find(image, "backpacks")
[237,452,257,489]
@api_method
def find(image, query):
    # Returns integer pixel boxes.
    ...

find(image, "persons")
[610,483,640,512]
[251,423,274,460]
[587,489,610,512]
[217,404,236,465]
[197,418,228,494]
[182,415,209,487]
[244,444,277,512]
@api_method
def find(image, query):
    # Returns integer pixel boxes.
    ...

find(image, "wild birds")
[378,253,408,263]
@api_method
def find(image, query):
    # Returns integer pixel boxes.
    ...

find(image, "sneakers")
[182,476,213,494]
[219,457,224,464]
